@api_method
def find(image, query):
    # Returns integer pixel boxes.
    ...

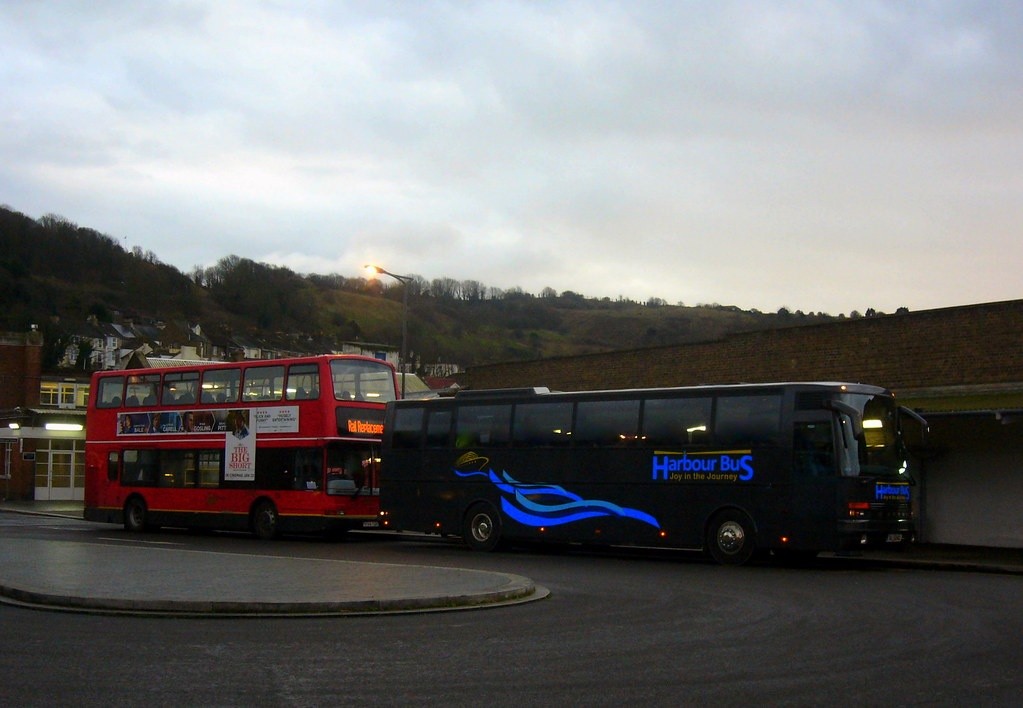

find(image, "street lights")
[363,263,416,399]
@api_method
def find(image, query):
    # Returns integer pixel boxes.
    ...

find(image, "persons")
[118,411,249,438]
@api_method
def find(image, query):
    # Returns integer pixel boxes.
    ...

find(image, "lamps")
[8,419,21,431]
[45,420,84,432]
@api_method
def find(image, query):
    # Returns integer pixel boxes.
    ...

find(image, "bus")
[82,352,403,542]
[377,384,937,570]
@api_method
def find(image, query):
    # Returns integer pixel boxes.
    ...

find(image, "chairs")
[113,387,365,406]
